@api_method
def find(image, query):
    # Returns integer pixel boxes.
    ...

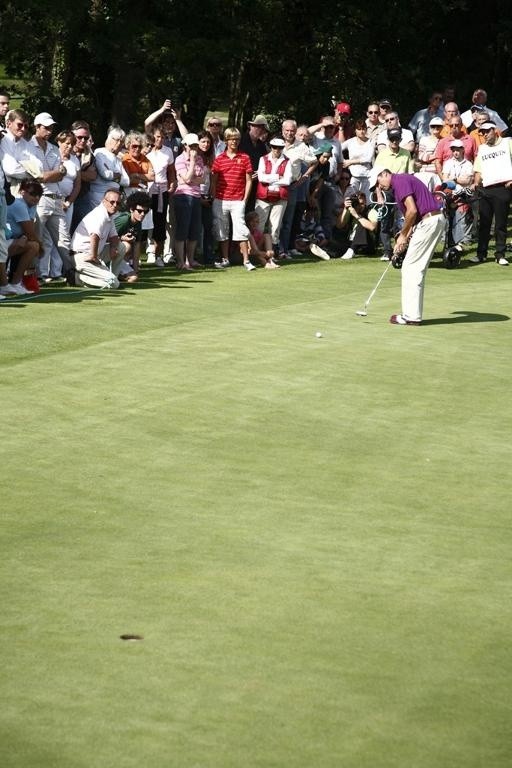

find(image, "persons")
[368,165,447,326]
[1,88,512,297]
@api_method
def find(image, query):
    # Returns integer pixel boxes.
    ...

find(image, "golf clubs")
[354,254,397,316]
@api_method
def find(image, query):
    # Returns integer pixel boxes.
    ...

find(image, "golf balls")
[315,332,321,337]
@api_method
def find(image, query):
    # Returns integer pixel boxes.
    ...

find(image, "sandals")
[190,261,206,268]
[175,264,194,270]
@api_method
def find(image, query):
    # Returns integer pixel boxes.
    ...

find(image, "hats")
[247,115,270,133]
[430,104,497,150]
[270,138,286,146]
[181,133,199,147]
[387,128,402,140]
[335,102,351,115]
[367,165,387,192]
[379,101,392,109]
[33,111,57,127]
[313,142,333,156]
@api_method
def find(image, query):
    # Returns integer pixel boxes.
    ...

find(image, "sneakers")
[243,261,256,270]
[147,254,176,267]
[0,285,25,300]
[390,314,421,326]
[380,253,390,261]
[309,242,330,260]
[495,257,509,266]
[468,256,488,263]
[264,250,303,268]
[340,248,355,259]
[12,285,35,295]
[221,258,230,266]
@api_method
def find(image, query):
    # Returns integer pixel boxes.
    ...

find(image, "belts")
[42,193,63,200]
[74,250,83,254]
[485,183,505,188]
[419,211,442,220]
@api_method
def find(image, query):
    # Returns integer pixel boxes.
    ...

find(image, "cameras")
[127,227,142,242]
[346,194,359,209]
[338,113,346,121]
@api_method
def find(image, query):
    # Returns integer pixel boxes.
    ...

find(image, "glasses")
[17,123,29,129]
[146,143,155,147]
[77,136,89,141]
[133,144,142,149]
[271,146,284,149]
[28,191,42,197]
[106,199,121,206]
[385,118,394,122]
[209,122,222,127]
[135,209,149,214]
[369,111,378,114]
